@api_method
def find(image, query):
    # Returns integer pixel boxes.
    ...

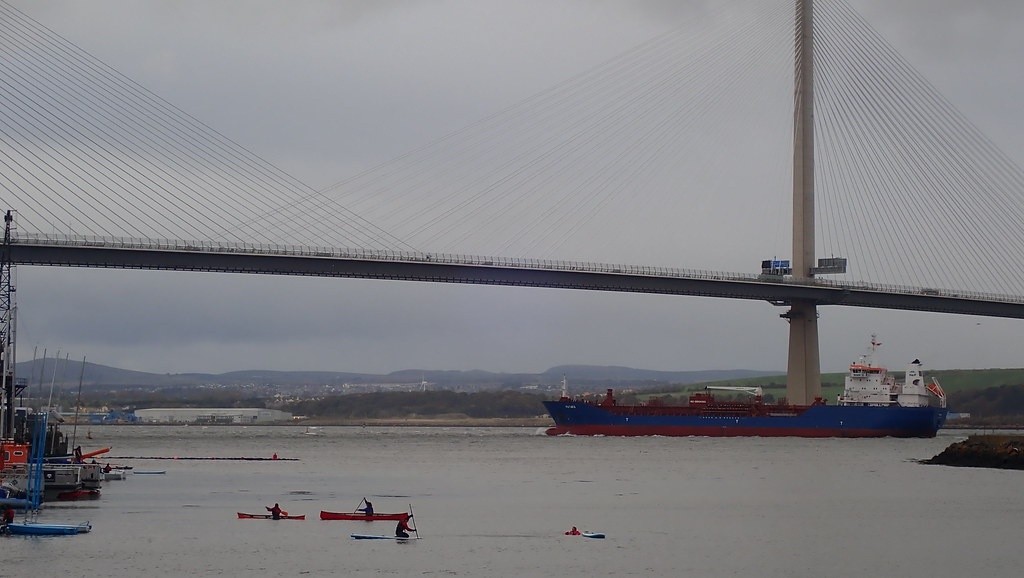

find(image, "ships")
[542,332,950,440]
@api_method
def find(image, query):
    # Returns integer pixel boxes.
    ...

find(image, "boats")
[3,521,92,536]
[237,512,306,520]
[319,509,410,521]
[134,470,166,474]
[582,530,607,538]
[350,533,423,540]
[56,491,101,501]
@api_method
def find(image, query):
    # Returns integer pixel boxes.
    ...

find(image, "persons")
[561,397,616,407]
[564,526,581,536]
[2,507,15,525]
[104,463,111,473]
[266,503,281,520]
[396,513,416,540]
[357,497,374,523]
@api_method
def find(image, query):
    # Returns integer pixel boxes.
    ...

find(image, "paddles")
[354,498,366,514]
[266,506,289,517]
[409,504,420,539]
